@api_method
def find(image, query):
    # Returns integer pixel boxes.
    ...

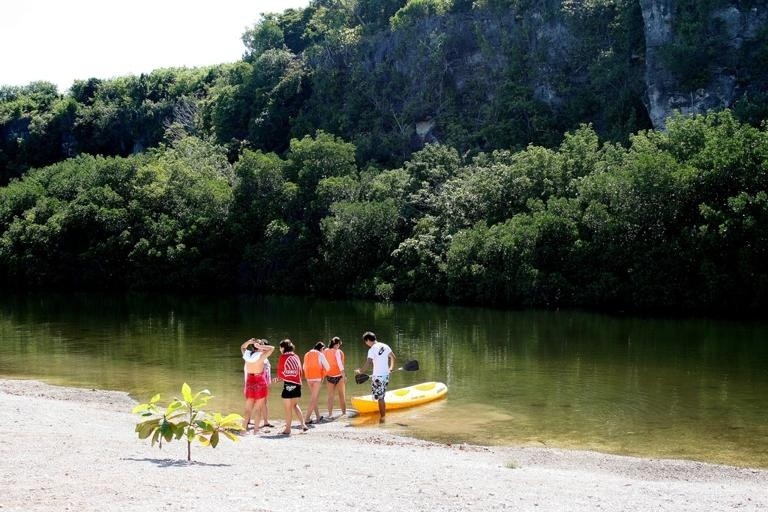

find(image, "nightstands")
[355,360,419,384]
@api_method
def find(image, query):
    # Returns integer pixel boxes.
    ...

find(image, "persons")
[303,341,331,424]
[237,337,275,436]
[322,337,349,418]
[354,332,396,424]
[272,339,309,435]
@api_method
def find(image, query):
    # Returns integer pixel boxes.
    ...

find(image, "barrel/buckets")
[264,423,274,427]
[319,415,323,422]
[303,428,307,432]
[279,431,290,434]
[305,420,315,424]
[248,423,255,425]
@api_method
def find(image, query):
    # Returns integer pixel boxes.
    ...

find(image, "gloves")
[351,382,448,414]
